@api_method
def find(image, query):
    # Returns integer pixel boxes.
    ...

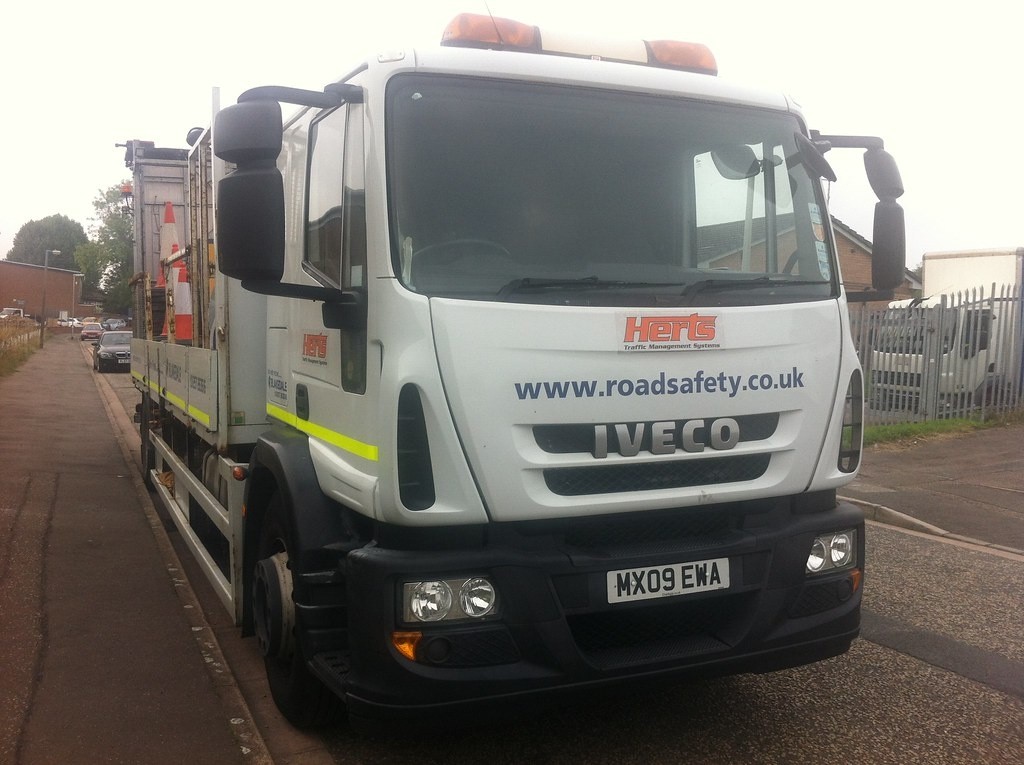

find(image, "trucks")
[114,13,906,725]
[869,248,1024,417]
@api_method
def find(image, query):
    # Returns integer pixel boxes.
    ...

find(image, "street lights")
[71,274,85,339]
[40,250,61,348]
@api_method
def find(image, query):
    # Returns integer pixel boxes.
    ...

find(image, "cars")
[82,316,100,326]
[91,331,133,372]
[81,324,106,341]
[102,318,126,330]
[57,318,85,328]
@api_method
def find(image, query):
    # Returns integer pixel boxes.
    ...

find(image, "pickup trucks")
[0,308,24,321]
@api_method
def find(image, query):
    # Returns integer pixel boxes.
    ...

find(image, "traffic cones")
[155,201,194,345]
